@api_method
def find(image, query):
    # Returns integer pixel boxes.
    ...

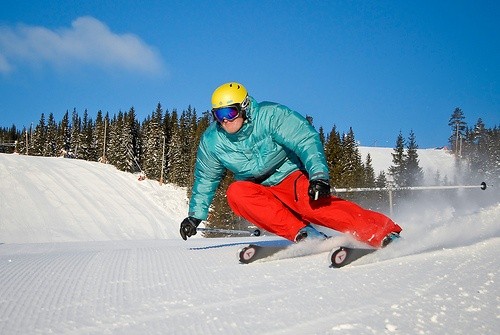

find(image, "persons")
[180,82,403,249]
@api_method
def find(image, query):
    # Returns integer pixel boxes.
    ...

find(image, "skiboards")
[239,236,384,269]
